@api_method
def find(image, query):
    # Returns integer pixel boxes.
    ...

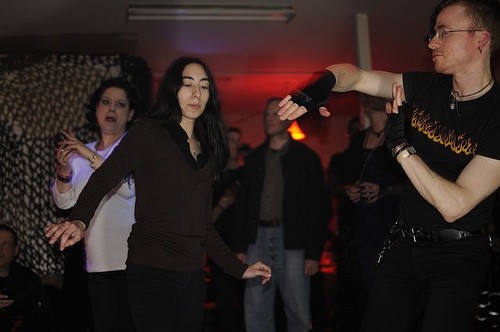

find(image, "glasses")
[428,28,481,42]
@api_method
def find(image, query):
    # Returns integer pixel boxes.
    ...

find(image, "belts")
[256,218,284,228]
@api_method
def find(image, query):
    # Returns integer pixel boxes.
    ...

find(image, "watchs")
[396,147,416,162]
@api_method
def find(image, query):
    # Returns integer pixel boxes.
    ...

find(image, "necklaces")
[450,77,493,110]
[188,138,196,156]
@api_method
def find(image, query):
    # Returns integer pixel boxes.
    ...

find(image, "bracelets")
[57,169,73,183]
[91,153,97,168]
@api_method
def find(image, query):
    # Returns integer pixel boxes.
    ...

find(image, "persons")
[53,77,137,332]
[211,127,252,332]
[0,224,55,332]
[328,97,404,332]
[238,97,331,332]
[278,0,500,332]
[44,56,271,332]
